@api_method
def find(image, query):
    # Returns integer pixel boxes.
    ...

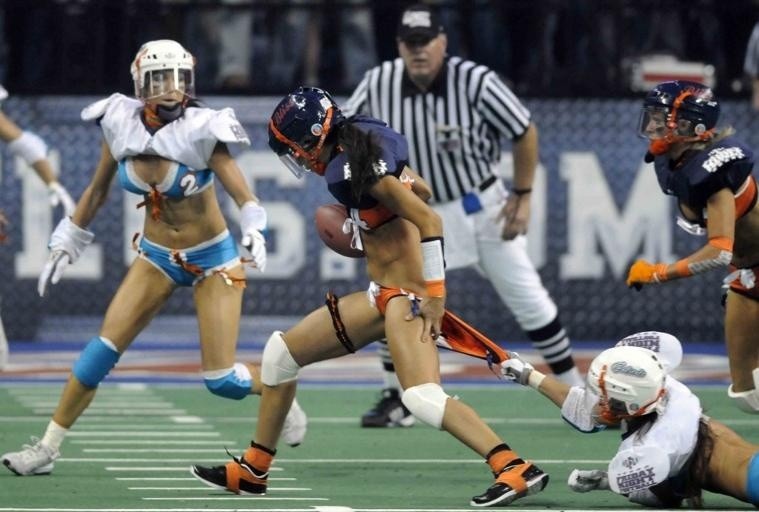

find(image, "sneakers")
[0,436,56,475]
[280,399,307,447]
[189,458,270,497]
[471,461,549,506]
[362,386,415,428]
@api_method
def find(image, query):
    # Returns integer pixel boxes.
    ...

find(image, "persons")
[501,331,759,511]
[0,86,75,374]
[332,8,605,433]
[0,40,306,476]
[188,86,549,507]
[628,82,759,413]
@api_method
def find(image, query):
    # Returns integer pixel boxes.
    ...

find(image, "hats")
[401,5,440,40]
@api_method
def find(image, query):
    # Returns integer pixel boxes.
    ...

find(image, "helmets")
[583,346,666,416]
[130,39,196,121]
[269,86,343,178]
[637,81,719,142]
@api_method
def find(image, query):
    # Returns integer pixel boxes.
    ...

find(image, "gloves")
[499,352,544,389]
[568,469,609,491]
[628,260,665,289]
[42,183,77,216]
[241,202,267,272]
[37,216,94,296]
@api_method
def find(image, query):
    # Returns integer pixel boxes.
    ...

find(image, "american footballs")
[315,203,367,258]
